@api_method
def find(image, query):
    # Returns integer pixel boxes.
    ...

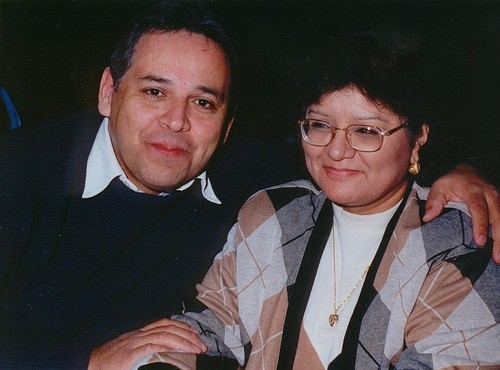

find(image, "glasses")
[296,116,410,153]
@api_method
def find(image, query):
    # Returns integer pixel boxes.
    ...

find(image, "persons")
[0,0,500,370]
[136,32,500,370]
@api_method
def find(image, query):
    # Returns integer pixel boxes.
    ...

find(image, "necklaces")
[329,224,370,327]
